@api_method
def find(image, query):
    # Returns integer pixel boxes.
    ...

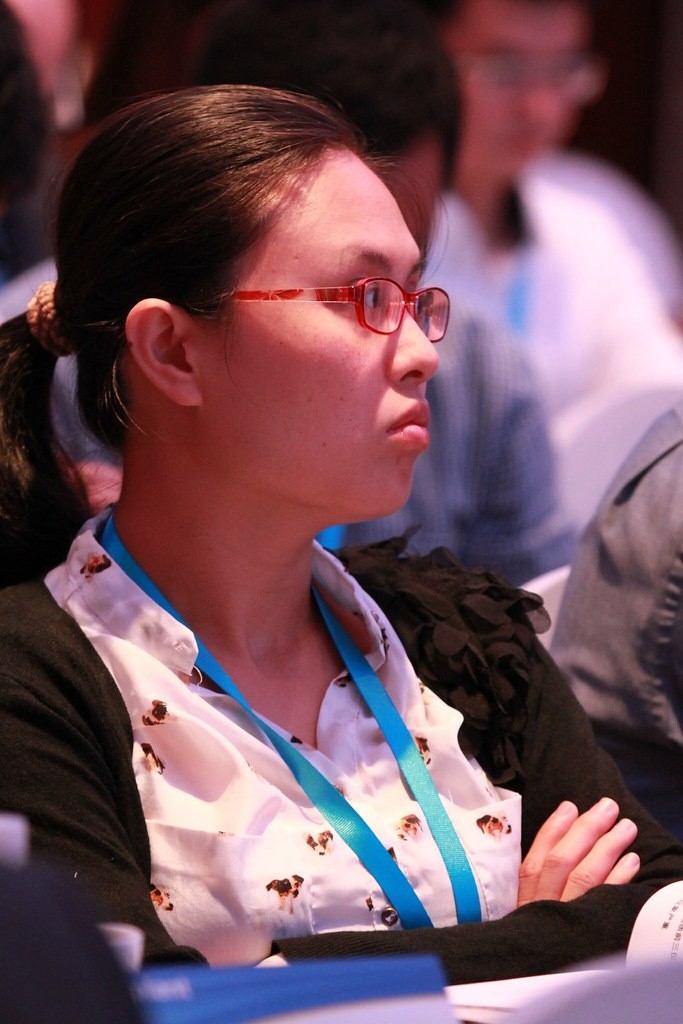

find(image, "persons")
[0,0,683,853]
[1,85,683,990]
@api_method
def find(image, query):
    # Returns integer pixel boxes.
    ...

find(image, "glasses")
[230,276,451,344]
